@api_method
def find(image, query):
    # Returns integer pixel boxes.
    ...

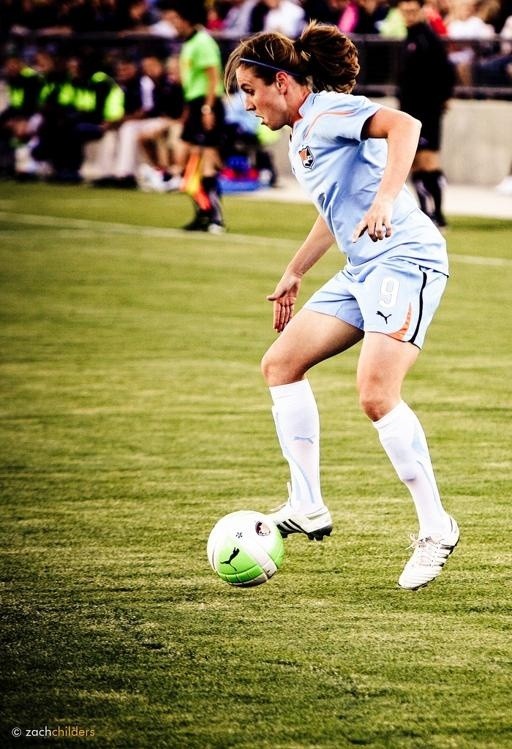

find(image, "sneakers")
[398,515,461,589]
[252,499,333,542]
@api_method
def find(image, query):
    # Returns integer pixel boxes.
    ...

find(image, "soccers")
[207,510,285,586]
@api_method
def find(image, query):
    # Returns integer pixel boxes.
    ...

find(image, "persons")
[223,18,461,592]
